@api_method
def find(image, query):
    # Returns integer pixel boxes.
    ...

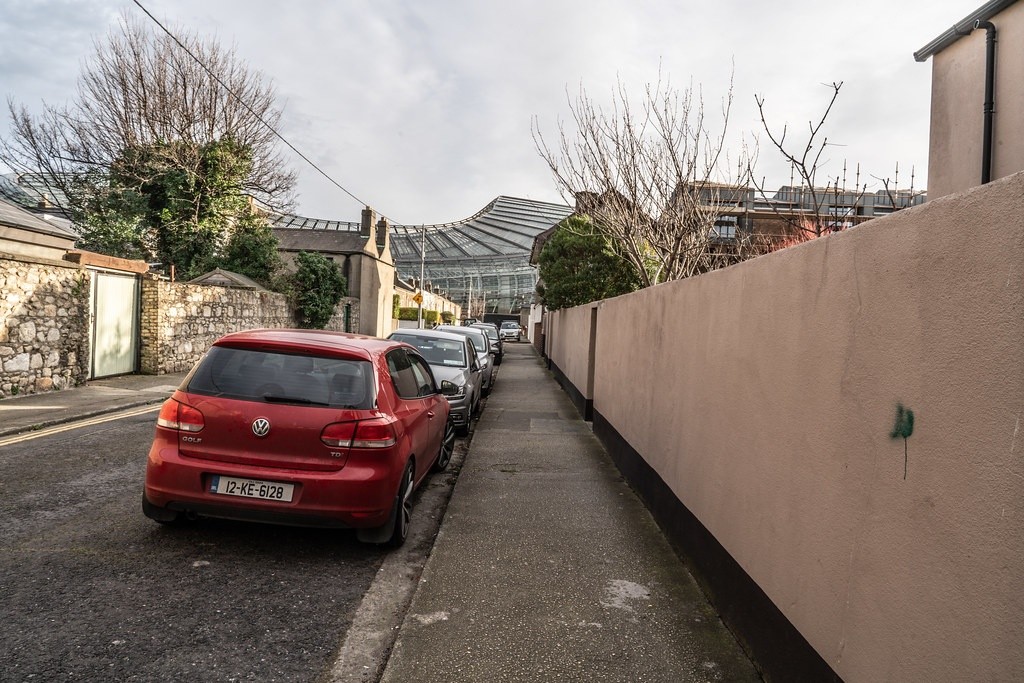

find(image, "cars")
[432,318,502,398]
[142,328,456,550]
[499,321,524,343]
[386,329,483,437]
